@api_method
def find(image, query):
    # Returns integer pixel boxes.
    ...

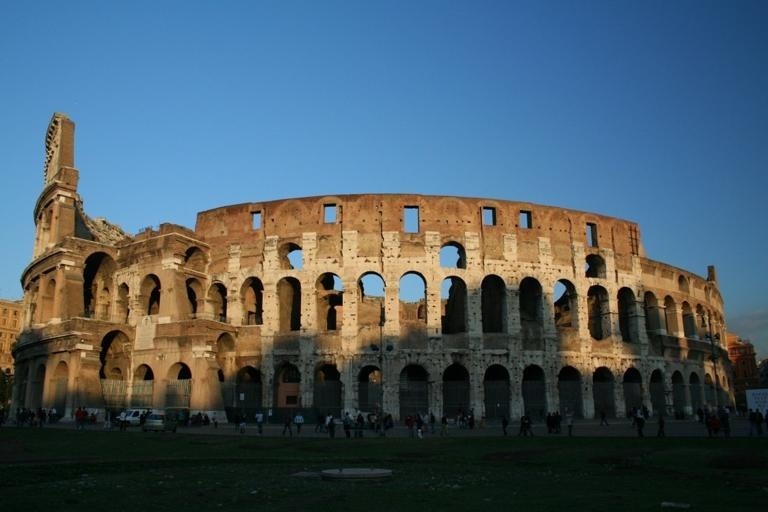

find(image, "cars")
[143,413,176,433]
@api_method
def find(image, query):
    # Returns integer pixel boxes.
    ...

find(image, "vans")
[116,408,148,426]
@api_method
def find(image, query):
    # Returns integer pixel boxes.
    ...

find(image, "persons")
[516,403,768,440]
[1,406,477,441]
[502,415,509,436]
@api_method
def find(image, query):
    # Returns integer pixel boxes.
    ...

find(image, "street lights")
[705,332,720,407]
[370,344,393,414]
[4,368,10,421]
